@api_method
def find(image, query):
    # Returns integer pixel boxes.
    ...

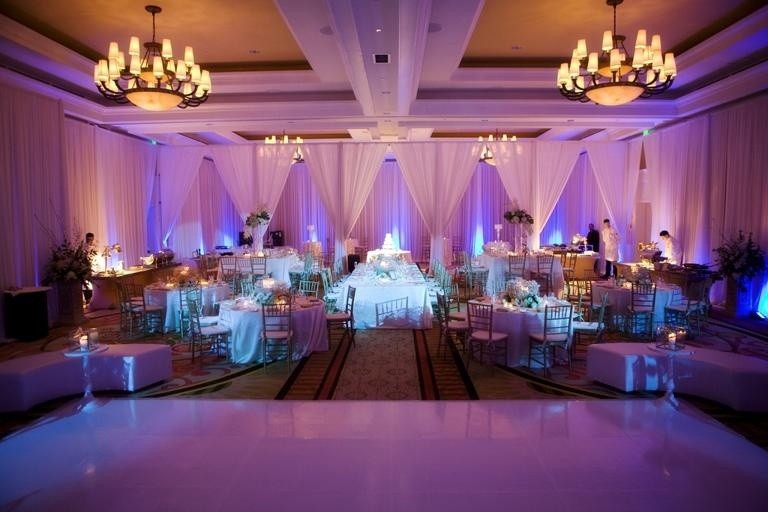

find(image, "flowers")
[502,206,534,226]
[244,202,271,227]
[34,197,100,288]
[707,222,767,291]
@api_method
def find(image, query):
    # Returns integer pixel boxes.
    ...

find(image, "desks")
[87,259,183,310]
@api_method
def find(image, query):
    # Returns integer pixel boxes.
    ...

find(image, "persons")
[659,230,683,266]
[81,233,99,252]
[599,219,621,282]
[586,223,599,273]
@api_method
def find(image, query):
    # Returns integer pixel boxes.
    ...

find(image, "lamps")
[554,1,677,106]
[94,5,212,113]
